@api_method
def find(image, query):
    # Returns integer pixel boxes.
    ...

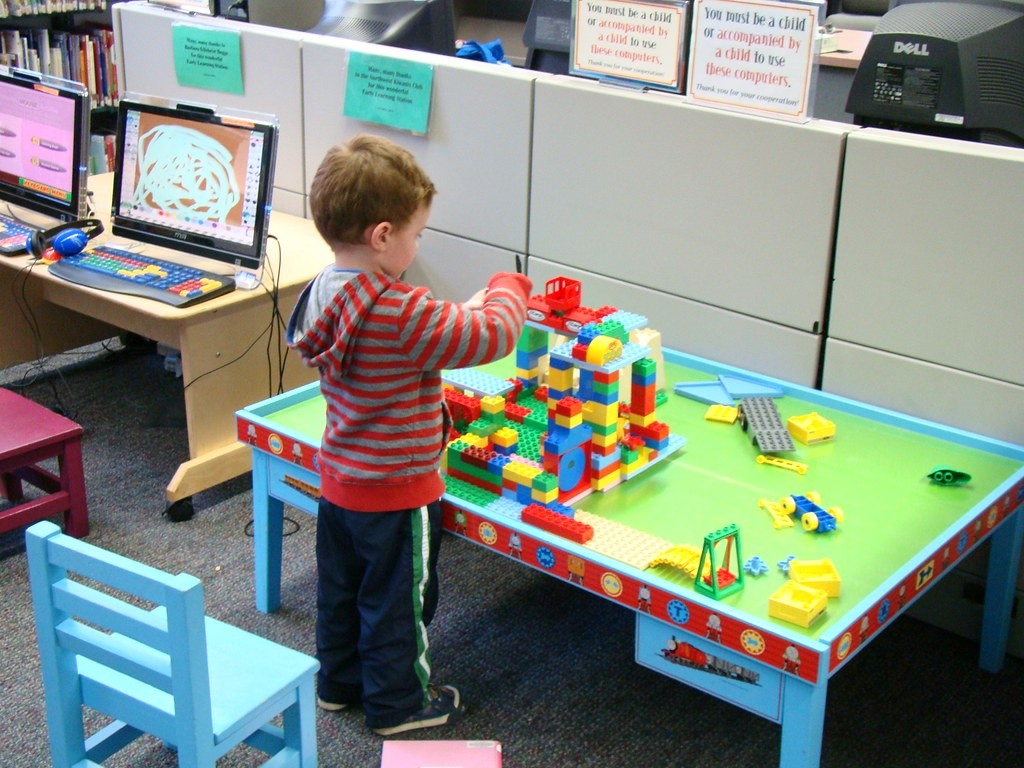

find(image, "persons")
[285,133,533,735]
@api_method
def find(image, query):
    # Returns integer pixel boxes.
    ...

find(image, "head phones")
[26,218,105,260]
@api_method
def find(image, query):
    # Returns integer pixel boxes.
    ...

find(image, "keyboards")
[0,212,48,257]
[48,245,237,308]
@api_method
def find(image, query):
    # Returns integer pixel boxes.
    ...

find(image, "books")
[381,740,503,768]
[0,0,119,175]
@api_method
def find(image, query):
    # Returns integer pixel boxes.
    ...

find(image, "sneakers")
[366,683,468,735]
[316,698,346,710]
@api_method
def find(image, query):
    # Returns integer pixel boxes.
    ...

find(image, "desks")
[234,320,1024,768]
[818,28,873,124]
[0,170,336,522]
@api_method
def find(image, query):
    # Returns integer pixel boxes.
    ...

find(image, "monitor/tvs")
[303,0,456,56]
[109,93,280,291]
[845,0,1024,149]
[0,65,91,223]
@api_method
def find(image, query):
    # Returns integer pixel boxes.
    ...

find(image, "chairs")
[0,387,90,538]
[26,520,320,768]
